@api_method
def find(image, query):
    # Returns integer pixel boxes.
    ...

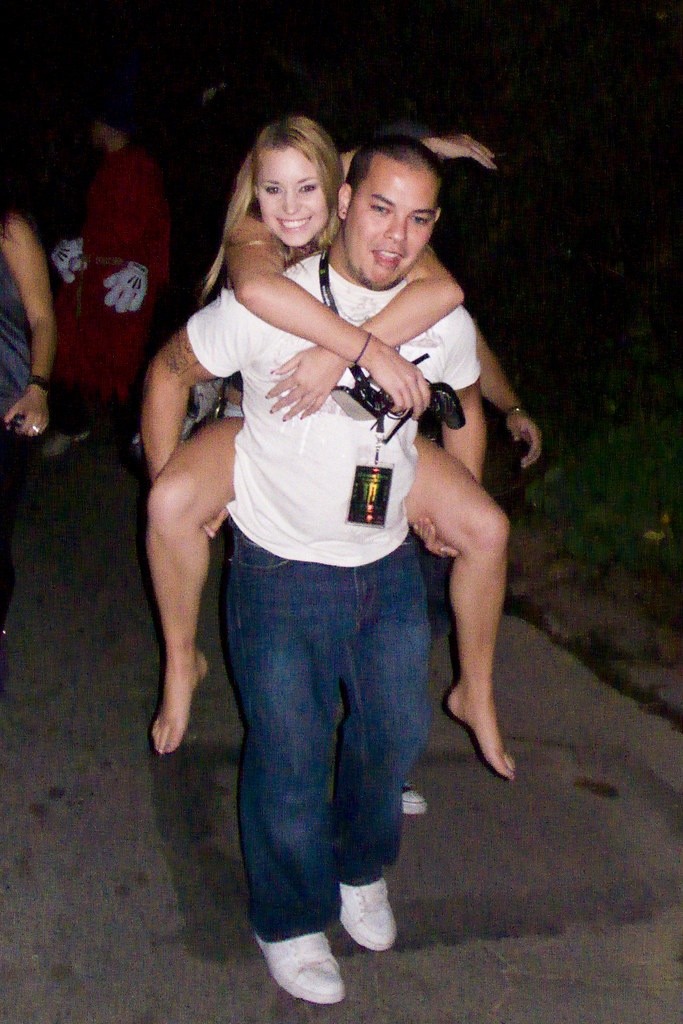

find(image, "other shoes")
[402,782,428,815]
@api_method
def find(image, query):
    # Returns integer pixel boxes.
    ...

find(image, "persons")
[0,203,57,694]
[39,104,172,456]
[141,113,543,1003]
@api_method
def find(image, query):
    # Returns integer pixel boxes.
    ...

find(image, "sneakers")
[252,930,346,1005]
[338,877,398,951]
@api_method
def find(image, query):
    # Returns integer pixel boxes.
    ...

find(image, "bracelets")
[355,333,373,363]
[508,406,525,414]
[28,375,49,393]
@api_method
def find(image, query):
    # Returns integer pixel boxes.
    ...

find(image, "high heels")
[329,353,466,433]
